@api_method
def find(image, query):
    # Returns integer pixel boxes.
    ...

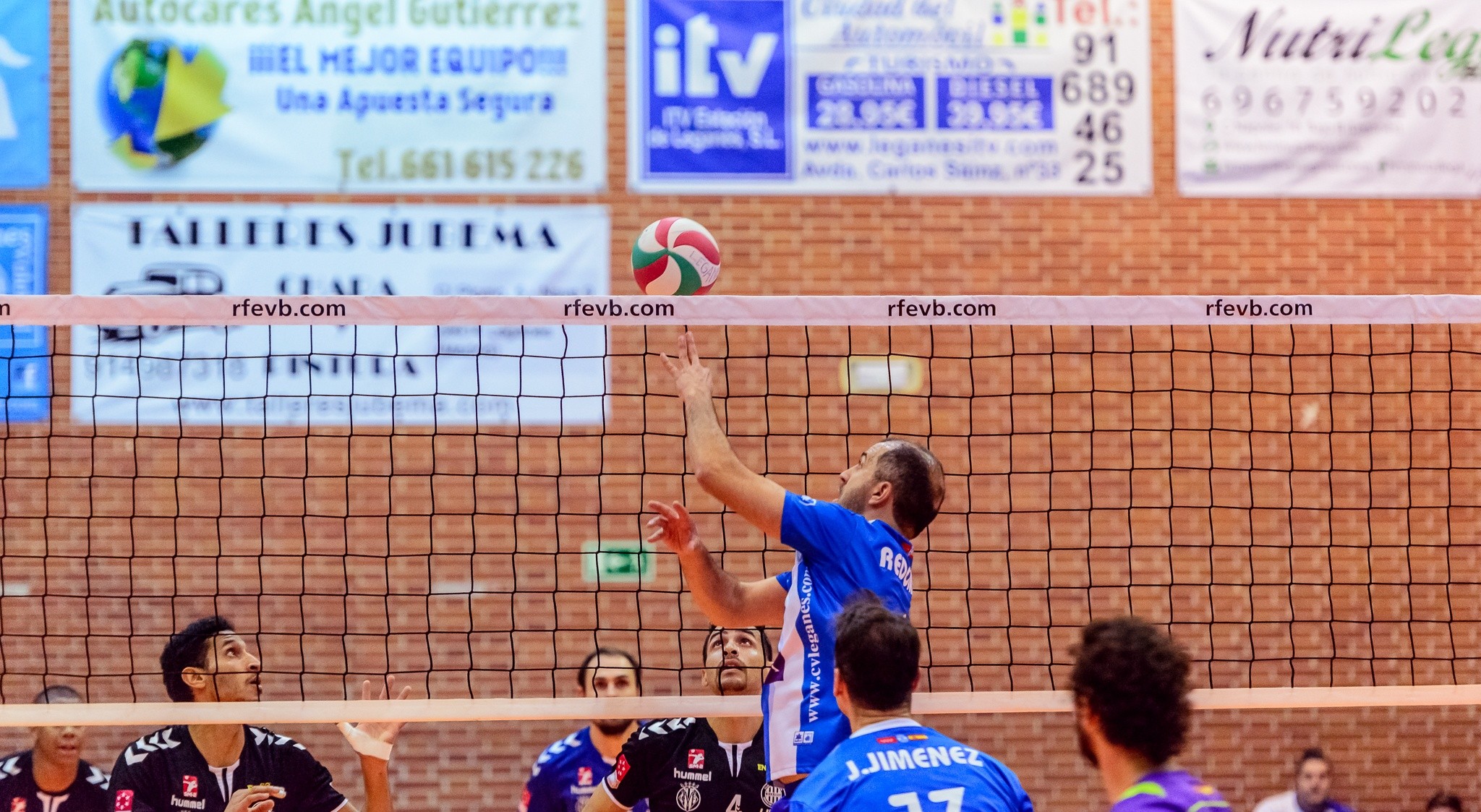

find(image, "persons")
[644,332,951,811]
[0,596,1465,812]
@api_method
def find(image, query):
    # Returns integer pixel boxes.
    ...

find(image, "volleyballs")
[630,217,722,296]
